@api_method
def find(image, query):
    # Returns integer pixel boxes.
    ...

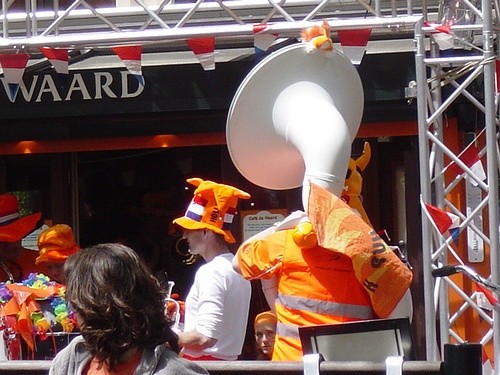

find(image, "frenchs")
[226,42,412,326]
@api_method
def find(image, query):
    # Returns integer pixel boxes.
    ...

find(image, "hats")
[173,178,251,243]
[0,193,44,243]
[254,310,277,326]
[34,224,80,264]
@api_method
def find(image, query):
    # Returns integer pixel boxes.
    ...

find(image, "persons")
[254,311,277,360]
[163,178,252,361]
[49,244,211,375]
[231,140,374,362]
[35,224,81,283]
[0,195,41,282]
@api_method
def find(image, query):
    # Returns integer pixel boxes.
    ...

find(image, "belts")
[182,354,223,361]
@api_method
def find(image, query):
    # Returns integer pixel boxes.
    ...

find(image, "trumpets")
[162,280,182,333]
